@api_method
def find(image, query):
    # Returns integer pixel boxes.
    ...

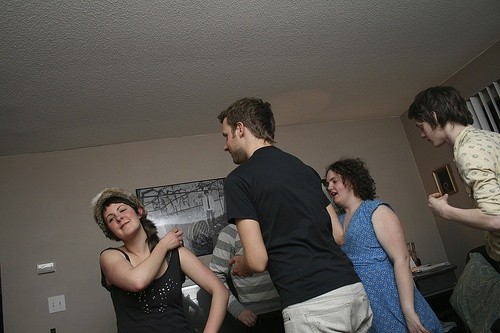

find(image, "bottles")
[408,241,421,268]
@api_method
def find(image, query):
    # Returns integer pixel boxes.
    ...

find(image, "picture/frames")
[136,177,228,257]
[432,164,458,196]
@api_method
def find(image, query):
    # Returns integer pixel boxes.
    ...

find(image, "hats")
[91,187,148,241]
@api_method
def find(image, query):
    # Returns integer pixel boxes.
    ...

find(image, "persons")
[407,85,500,280]
[207,222,285,333]
[324,155,446,333]
[214,96,374,333]
[92,187,233,333]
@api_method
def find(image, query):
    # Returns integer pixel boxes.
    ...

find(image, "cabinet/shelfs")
[413,265,466,333]
